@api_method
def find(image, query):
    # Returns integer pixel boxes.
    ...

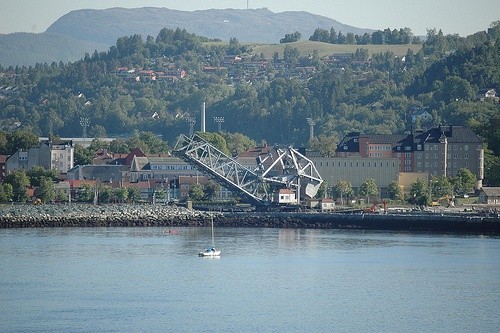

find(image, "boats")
[199,216,221,257]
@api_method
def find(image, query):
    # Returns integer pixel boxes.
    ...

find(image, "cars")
[431,197,447,205]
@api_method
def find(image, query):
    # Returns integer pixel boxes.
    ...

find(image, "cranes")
[172,131,323,204]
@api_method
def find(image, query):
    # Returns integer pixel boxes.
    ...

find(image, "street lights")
[439,134,448,179]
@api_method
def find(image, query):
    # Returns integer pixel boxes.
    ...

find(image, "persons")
[206,248,211,253]
[211,248,216,253]
